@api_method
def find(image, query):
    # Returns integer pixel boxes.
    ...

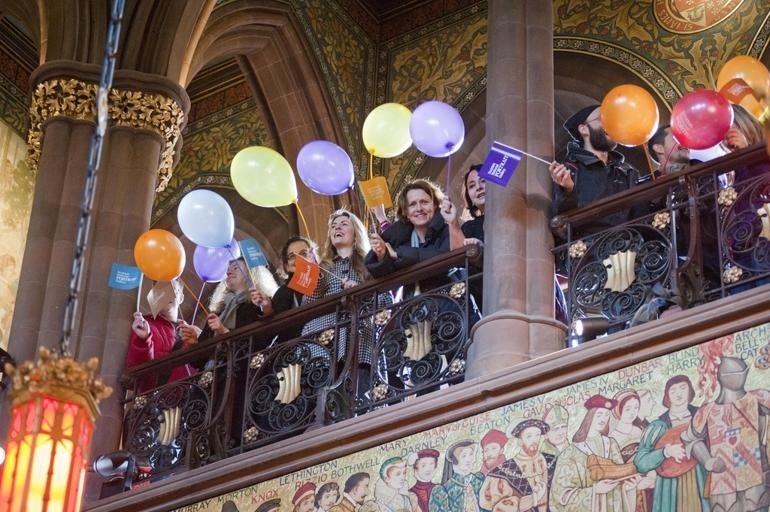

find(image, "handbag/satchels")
[120,406,161,448]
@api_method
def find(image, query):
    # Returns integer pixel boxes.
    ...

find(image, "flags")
[478,143,523,187]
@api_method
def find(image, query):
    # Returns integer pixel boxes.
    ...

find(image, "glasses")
[285,251,307,260]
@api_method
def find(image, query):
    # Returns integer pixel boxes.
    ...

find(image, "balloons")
[297,140,355,195]
[362,103,413,159]
[409,100,464,158]
[600,84,660,149]
[230,146,299,208]
[670,87,734,150]
[717,55,770,119]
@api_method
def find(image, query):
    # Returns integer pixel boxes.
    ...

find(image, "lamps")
[94,450,135,492]
[0,344,114,512]
[576,317,631,336]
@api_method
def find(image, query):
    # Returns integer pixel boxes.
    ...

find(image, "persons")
[549,105,769,343]
[113,163,571,478]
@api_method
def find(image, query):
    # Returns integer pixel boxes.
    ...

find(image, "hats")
[415,448,439,458]
[222,500,239,512]
[512,419,551,436]
[292,483,316,505]
[481,429,508,447]
[564,104,600,140]
[612,388,636,419]
[543,405,569,429]
[584,394,618,410]
[255,498,280,512]
